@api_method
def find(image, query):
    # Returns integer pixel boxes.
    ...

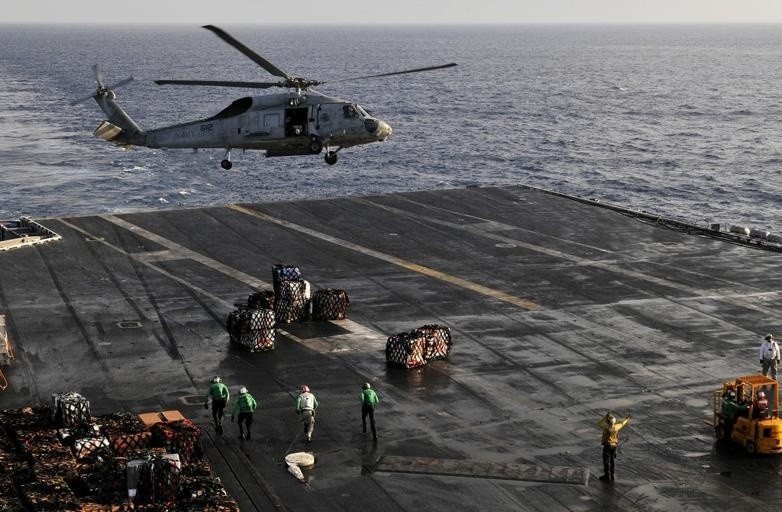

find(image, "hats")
[758,392,765,398]
[765,334,774,340]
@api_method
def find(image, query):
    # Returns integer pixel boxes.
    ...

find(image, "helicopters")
[70,25,458,170]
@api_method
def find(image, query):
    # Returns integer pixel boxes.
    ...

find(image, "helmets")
[728,390,736,399]
[607,415,616,425]
[300,385,310,394]
[239,386,248,394]
[362,382,371,390]
[212,376,220,383]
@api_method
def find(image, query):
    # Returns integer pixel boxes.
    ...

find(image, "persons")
[722,391,747,421]
[205,376,229,433]
[296,385,318,442]
[754,391,769,418]
[760,333,780,381]
[231,387,257,441]
[360,382,379,440]
[597,411,633,480]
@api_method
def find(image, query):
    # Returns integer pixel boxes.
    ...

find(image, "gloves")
[204,403,209,410]
[777,360,780,364]
[296,410,300,415]
[760,359,763,364]
[626,414,633,419]
[224,400,228,408]
[605,411,610,419]
[231,415,235,423]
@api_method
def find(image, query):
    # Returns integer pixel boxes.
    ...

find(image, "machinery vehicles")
[703,373,782,455]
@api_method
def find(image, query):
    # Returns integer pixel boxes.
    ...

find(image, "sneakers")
[304,433,311,442]
[238,432,251,440]
[363,429,378,442]
[599,474,615,482]
[214,425,224,433]
